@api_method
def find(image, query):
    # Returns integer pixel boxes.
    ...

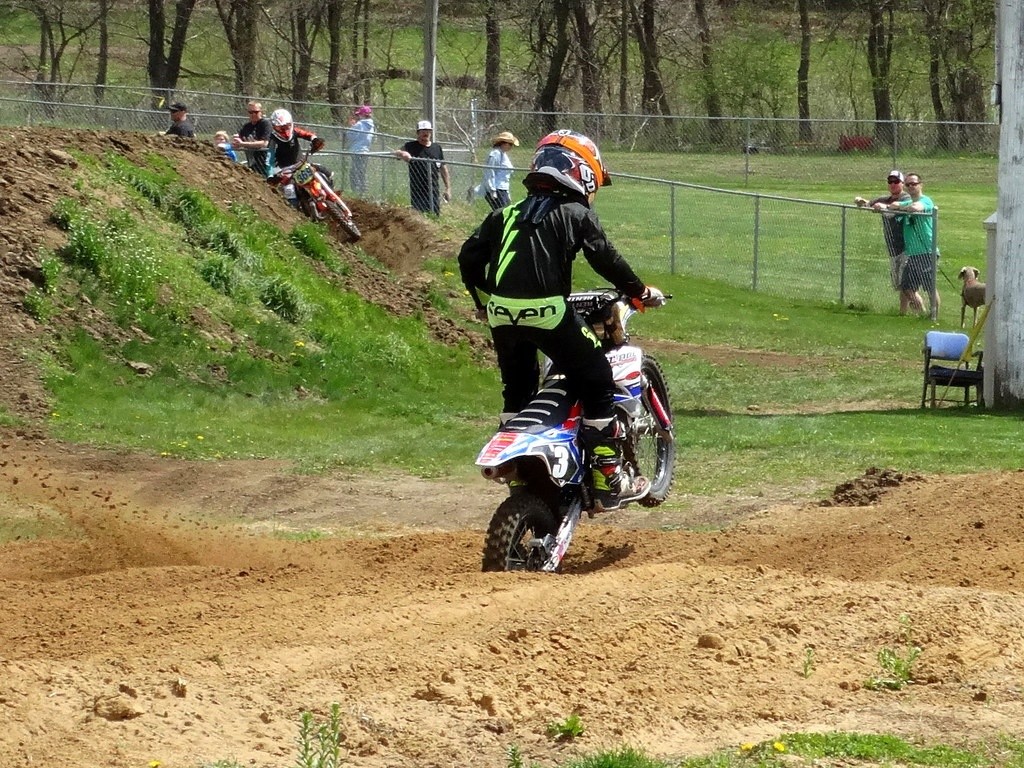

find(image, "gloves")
[312,138,325,152]
[642,285,666,309]
[267,177,282,186]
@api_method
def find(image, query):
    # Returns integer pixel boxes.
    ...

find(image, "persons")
[394,120,451,218]
[265,109,334,213]
[456,129,666,511]
[164,102,194,137]
[343,106,375,192]
[474,132,520,211]
[233,100,271,175]
[213,130,237,163]
[889,174,941,317]
[855,170,909,318]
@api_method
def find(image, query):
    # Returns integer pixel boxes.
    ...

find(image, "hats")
[887,171,904,182]
[355,107,371,116]
[417,120,433,130]
[494,131,520,147]
[168,102,188,111]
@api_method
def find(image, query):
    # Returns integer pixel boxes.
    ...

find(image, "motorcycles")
[275,142,362,243]
[475,287,678,573]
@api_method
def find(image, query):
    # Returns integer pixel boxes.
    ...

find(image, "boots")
[578,415,652,511]
[506,481,527,496]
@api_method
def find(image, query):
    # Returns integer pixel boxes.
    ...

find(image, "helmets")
[530,129,612,205]
[270,109,294,142]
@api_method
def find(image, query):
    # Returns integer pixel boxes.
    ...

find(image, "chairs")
[921,331,984,408]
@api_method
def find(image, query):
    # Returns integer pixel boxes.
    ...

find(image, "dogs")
[958,266,986,328]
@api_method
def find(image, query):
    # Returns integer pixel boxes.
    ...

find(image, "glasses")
[905,182,919,187]
[248,111,259,114]
[887,179,899,185]
